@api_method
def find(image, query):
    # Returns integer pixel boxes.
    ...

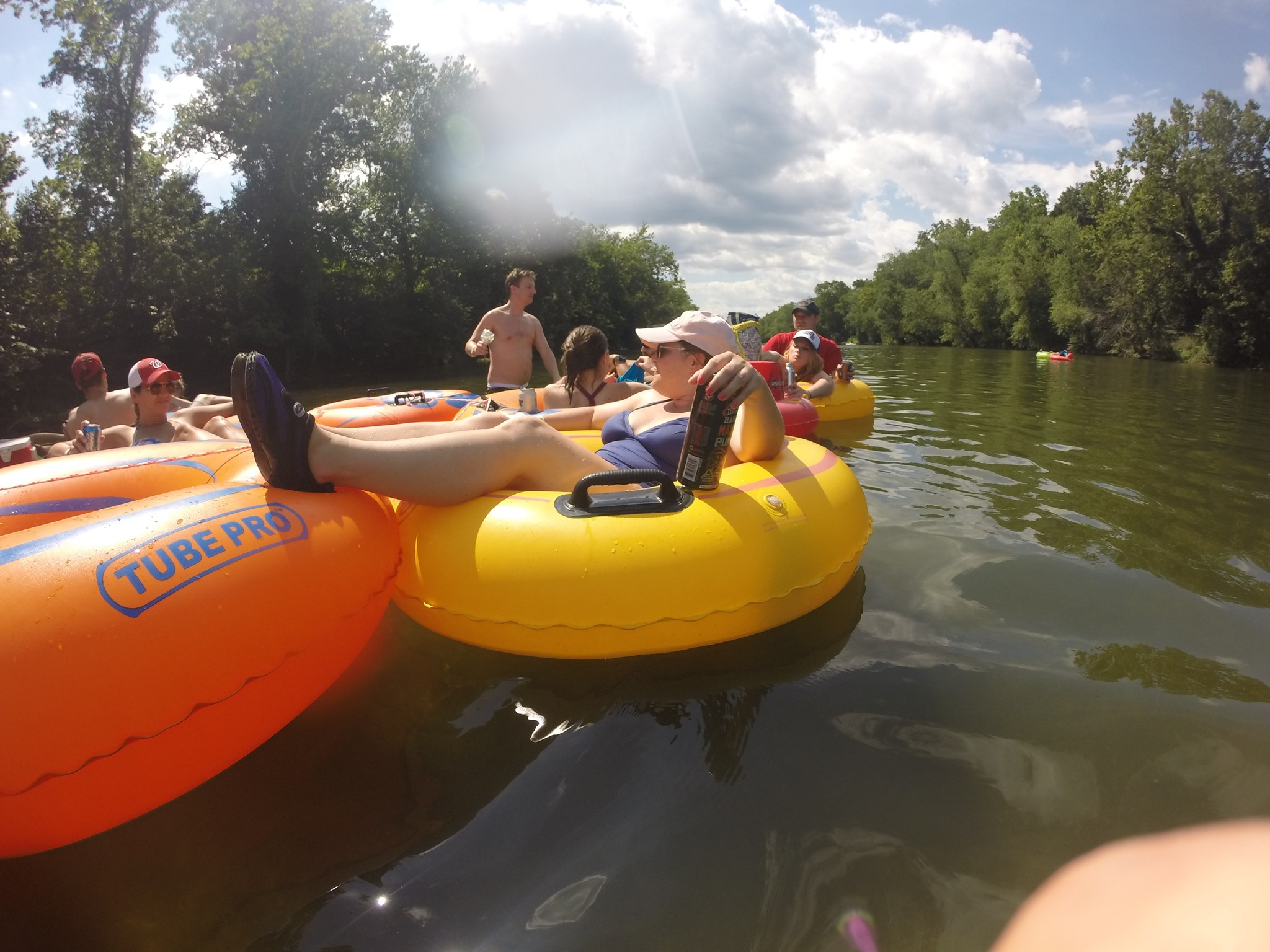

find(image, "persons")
[542,299,855,407]
[0,352,248,458]
[464,268,562,395]
[229,311,785,506]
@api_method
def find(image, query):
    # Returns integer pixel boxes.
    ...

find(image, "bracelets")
[806,390,812,399]
[64,448,70,455]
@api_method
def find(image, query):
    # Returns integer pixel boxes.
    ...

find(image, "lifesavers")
[774,395,820,438]
[1050,352,1073,361]
[0,438,404,862]
[1036,351,1050,360]
[307,386,482,428]
[393,427,872,665]
[793,378,875,422]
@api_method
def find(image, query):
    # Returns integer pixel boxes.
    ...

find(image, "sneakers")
[229,350,334,494]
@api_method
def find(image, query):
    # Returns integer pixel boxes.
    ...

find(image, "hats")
[792,299,820,315]
[72,352,102,382]
[126,357,181,390]
[792,329,821,351]
[635,310,739,357]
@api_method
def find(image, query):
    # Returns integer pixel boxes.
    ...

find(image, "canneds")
[839,360,852,381]
[83,423,102,452]
[676,373,739,490]
[519,388,537,413]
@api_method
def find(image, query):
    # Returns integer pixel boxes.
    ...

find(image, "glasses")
[147,382,176,396]
[656,344,683,358]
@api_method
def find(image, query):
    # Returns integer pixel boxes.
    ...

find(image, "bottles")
[784,363,795,392]
